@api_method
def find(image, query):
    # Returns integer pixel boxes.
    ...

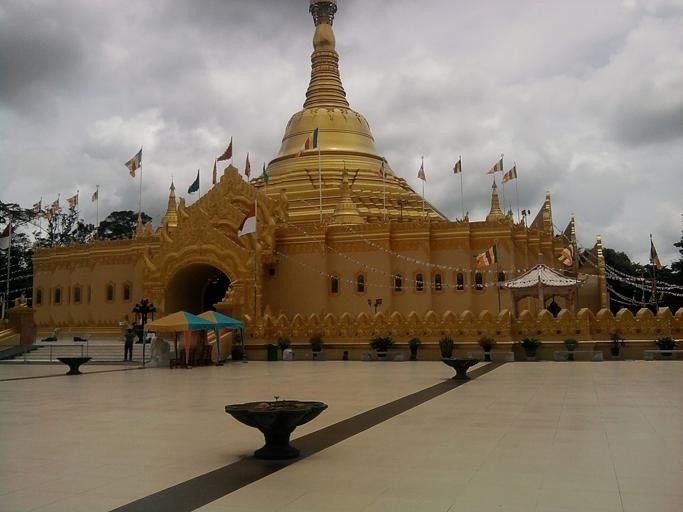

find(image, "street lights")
[132,298,157,367]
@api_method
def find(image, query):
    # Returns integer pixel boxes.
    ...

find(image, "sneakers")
[122,359,131,362]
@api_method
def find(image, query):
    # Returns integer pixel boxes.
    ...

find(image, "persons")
[123,329,135,363]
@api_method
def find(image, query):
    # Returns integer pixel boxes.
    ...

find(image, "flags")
[236,199,257,237]
[53,192,60,214]
[216,136,233,164]
[33,195,41,214]
[452,154,462,174]
[649,233,661,271]
[0,213,12,254]
[123,147,143,179]
[44,204,55,221]
[475,241,497,269]
[417,155,426,183]
[500,164,518,184]
[91,184,99,202]
[556,241,574,269]
[65,189,79,210]
[296,126,319,158]
[485,153,504,173]
[379,156,393,180]
[261,161,268,183]
[212,160,218,184]
[244,152,253,180]
[186,168,200,195]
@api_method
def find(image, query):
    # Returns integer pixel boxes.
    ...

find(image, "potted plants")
[519,336,541,360]
[480,336,495,361]
[439,336,454,362]
[408,338,421,360]
[565,338,577,360]
[608,333,622,360]
[278,335,290,358]
[370,337,395,360]
[310,336,321,360]
[657,335,680,359]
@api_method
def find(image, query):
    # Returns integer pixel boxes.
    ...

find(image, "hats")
[128,327,131,330]
